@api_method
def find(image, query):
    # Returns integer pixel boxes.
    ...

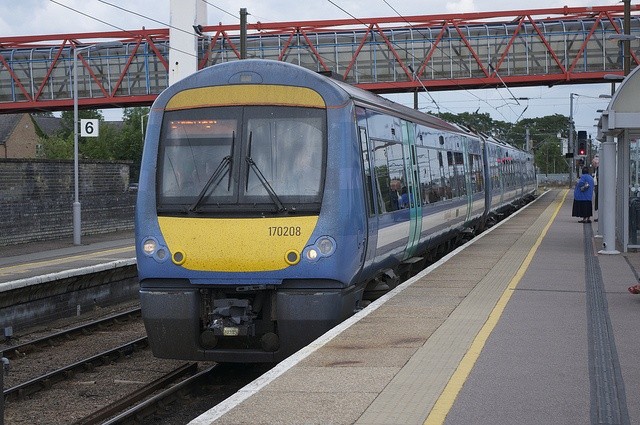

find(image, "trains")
[129,56,539,365]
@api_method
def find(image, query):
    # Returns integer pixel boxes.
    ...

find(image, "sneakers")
[587,219,592,223]
[578,220,586,223]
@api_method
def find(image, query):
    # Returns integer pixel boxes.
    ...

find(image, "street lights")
[72,41,123,245]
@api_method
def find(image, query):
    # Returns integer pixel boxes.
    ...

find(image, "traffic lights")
[579,141,586,155]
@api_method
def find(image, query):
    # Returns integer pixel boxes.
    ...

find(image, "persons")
[592,158,600,221]
[572,167,593,222]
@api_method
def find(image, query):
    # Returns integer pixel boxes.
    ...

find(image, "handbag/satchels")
[581,182,590,191]
